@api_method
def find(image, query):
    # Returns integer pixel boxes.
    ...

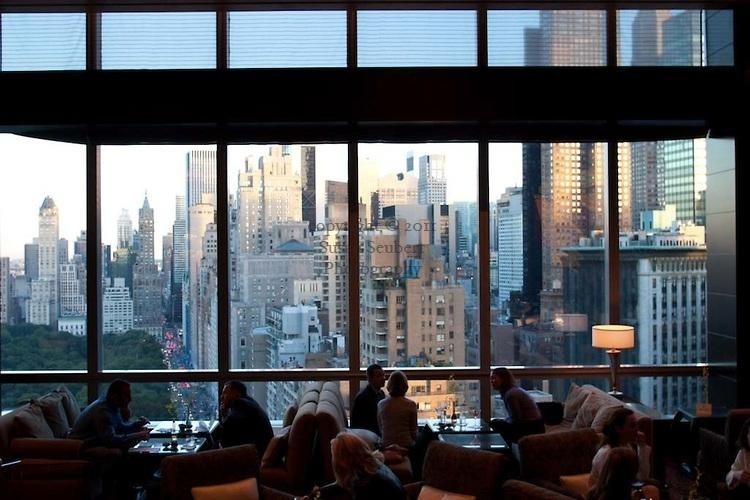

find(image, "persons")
[70,379,151,458]
[725,419,750,489]
[350,364,385,434]
[312,432,406,499]
[377,372,418,449]
[590,408,638,486]
[586,448,638,500]
[490,368,545,438]
[211,381,273,460]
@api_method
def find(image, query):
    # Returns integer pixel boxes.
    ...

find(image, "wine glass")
[468,409,482,428]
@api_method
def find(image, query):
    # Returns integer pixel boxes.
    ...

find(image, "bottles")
[185,407,192,427]
[170,419,177,449]
[450,400,458,424]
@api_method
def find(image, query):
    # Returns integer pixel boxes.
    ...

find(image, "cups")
[460,416,466,425]
[185,434,195,448]
[179,424,185,430]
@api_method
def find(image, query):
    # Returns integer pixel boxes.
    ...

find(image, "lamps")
[589,323,638,398]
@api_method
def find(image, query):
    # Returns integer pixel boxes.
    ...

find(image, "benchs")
[0,371,749,500]
